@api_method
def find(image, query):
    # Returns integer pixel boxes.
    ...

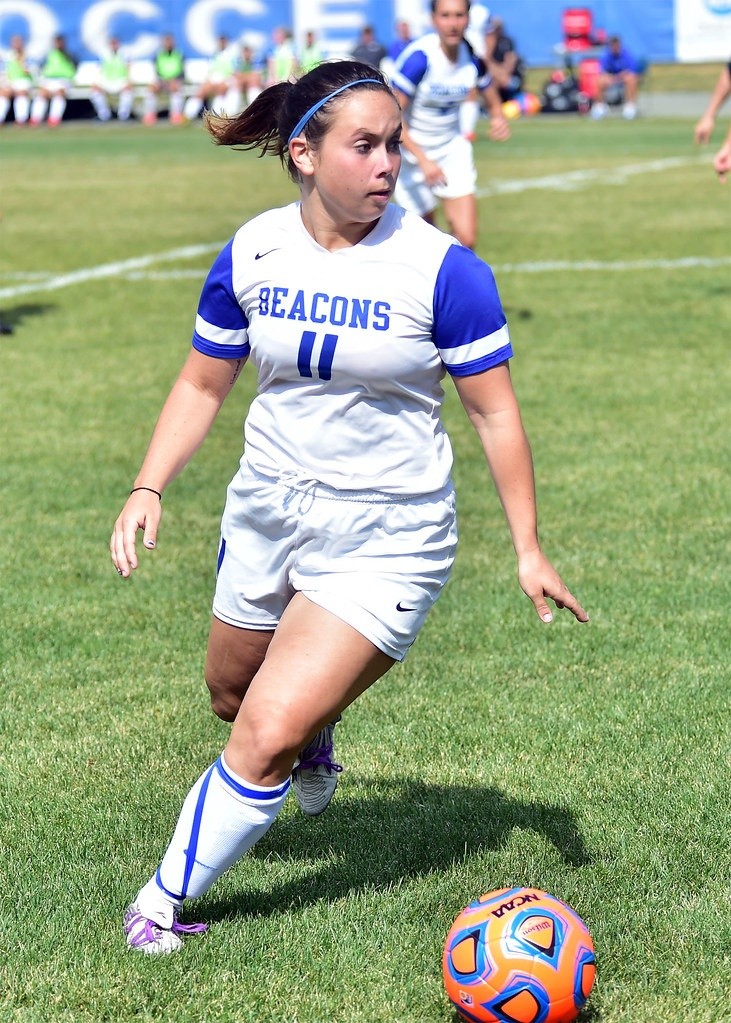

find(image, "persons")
[483,15,648,123]
[697,59,730,177]
[1,20,416,125]
[379,0,510,256]
[108,63,584,964]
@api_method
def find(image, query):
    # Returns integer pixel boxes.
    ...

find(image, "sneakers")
[291,714,344,817]
[121,896,186,956]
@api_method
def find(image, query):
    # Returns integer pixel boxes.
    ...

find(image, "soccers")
[440,882,598,1023]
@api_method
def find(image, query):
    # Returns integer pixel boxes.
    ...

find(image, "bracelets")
[128,486,163,498]
